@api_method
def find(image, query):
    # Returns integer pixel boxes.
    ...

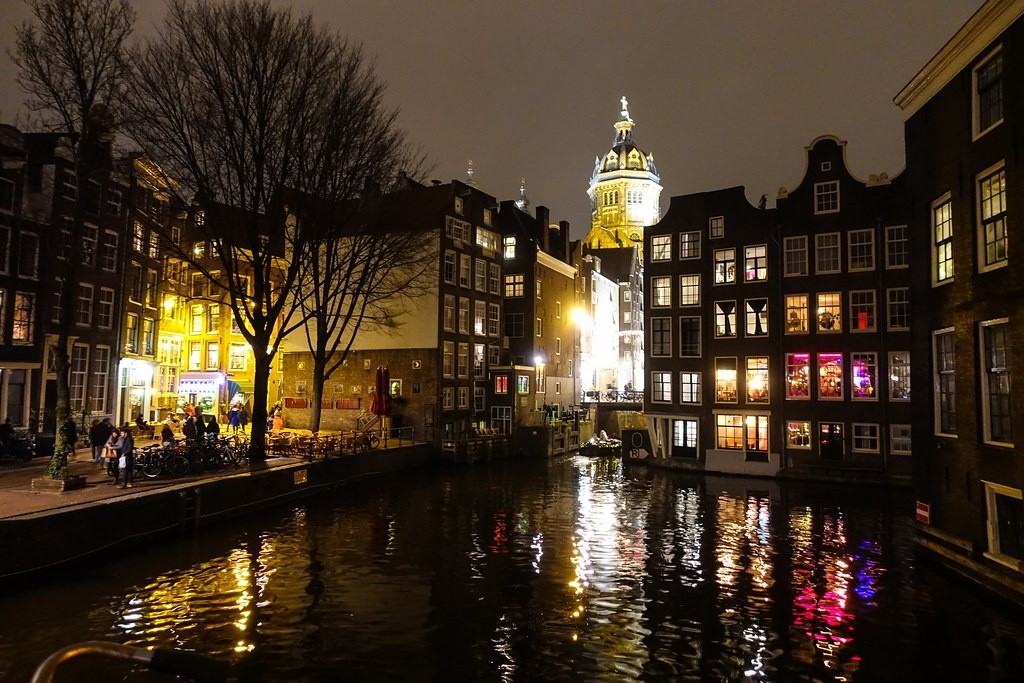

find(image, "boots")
[100,464,107,470]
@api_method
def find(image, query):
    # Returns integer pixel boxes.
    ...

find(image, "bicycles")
[103,427,381,478]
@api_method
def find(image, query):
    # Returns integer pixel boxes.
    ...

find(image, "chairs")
[263,429,362,459]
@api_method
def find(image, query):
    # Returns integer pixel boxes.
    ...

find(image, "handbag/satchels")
[119,453,127,469]
[101,444,118,458]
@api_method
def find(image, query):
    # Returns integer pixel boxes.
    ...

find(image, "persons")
[28,418,38,434]
[183,402,220,439]
[226,403,248,433]
[67,415,77,456]
[136,414,153,430]
[89,418,134,488]
[529,402,554,421]
[0,417,15,448]
[162,425,174,443]
[266,402,283,431]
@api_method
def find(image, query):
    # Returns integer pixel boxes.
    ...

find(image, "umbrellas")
[372,367,393,440]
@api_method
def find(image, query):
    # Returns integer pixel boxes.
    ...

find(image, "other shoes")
[117,481,133,489]
[91,459,96,462]
